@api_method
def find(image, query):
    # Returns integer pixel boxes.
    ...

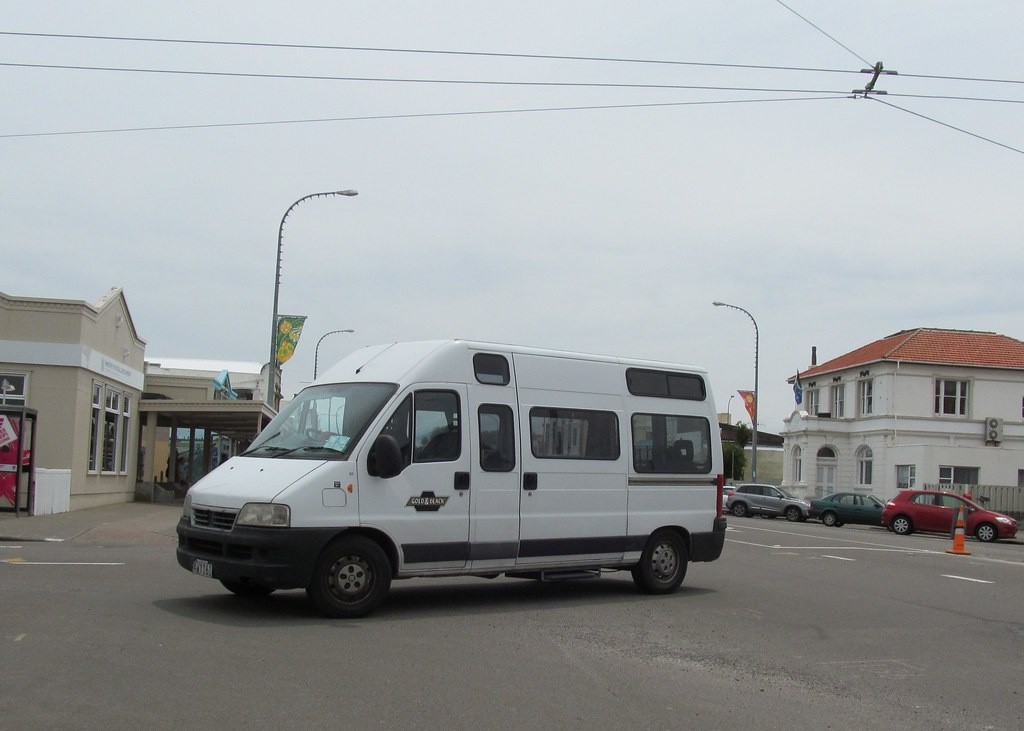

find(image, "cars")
[810,492,887,528]
[882,488,1019,540]
[722,485,736,513]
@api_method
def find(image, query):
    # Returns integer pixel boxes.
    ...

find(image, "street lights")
[727,395,735,425]
[713,300,759,483]
[267,190,359,410]
[313,329,354,381]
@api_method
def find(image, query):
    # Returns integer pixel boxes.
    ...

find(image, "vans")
[175,338,727,619]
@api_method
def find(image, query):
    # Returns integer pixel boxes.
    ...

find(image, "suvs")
[726,483,811,522]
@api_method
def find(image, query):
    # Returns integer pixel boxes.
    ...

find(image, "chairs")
[932,497,944,506]
[854,498,858,505]
[420,431,461,462]
[673,440,696,473]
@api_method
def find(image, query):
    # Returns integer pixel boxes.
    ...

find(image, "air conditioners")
[986,417,1003,429]
[985,429,1003,442]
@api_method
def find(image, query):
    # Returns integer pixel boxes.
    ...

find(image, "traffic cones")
[946,504,971,555]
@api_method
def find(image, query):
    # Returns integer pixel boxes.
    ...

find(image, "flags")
[277,317,305,365]
[793,370,802,404]
[738,390,755,425]
